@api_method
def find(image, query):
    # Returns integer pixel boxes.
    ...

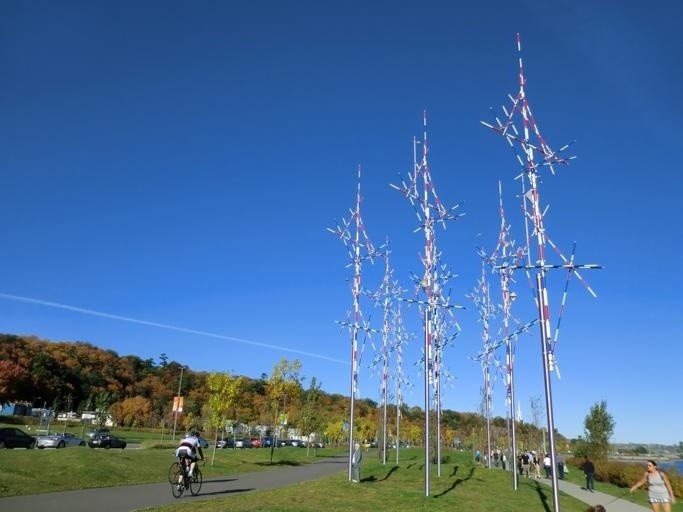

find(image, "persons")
[175,431,206,493]
[582,455,596,490]
[351,442,362,484]
[473,446,567,479]
[628,459,676,512]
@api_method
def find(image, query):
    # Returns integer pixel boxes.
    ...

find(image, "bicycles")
[169,457,204,498]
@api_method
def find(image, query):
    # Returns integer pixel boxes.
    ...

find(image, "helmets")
[191,431,200,437]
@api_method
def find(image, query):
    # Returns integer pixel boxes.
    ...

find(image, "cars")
[36,433,86,449]
[0,427,38,449]
[217,438,323,448]
[88,434,127,449]
[199,437,209,448]
